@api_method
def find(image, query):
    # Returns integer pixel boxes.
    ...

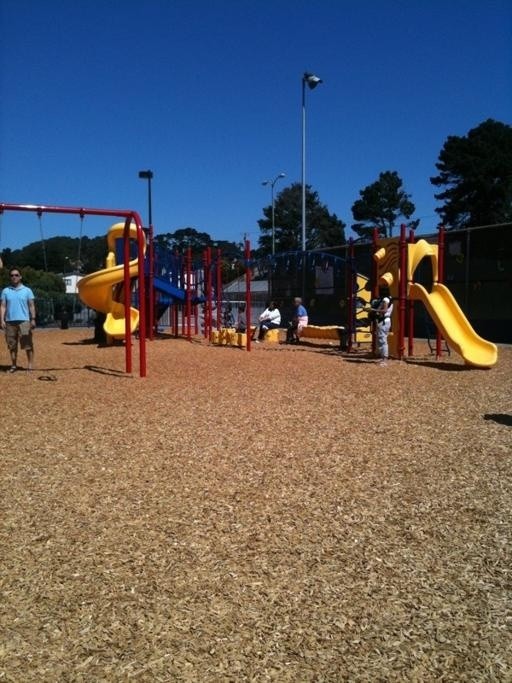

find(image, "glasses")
[10,274,19,277]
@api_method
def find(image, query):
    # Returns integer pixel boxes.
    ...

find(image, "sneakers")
[255,339,261,344]
[262,325,269,330]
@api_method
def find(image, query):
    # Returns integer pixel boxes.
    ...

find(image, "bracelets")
[31,318,36,320]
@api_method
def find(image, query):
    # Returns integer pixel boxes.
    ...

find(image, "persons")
[370,283,394,366]
[222,305,247,334]
[288,297,308,344]
[0,268,36,375]
[255,300,282,344]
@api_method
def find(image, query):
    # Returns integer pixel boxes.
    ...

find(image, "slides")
[76,223,147,340]
[407,282,498,369]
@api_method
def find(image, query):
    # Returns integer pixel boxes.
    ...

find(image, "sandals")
[9,366,17,373]
